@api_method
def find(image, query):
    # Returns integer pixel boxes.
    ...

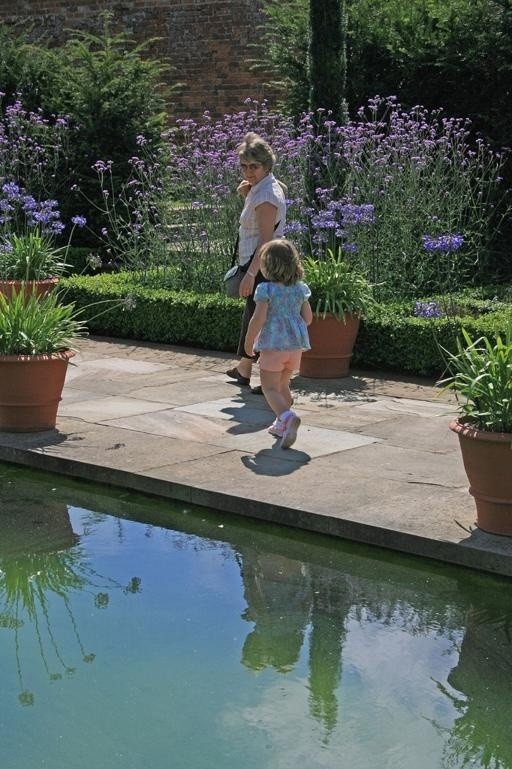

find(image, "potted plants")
[1,227,72,303]
[1,283,92,433]
[434,602,508,766]
[434,319,512,535]
[292,244,389,380]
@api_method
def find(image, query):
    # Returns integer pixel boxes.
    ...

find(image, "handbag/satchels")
[223,265,247,297]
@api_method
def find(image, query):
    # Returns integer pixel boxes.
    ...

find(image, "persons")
[226,132,286,395]
[243,240,313,449]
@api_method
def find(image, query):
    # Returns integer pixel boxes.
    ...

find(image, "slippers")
[225,366,250,384]
[249,386,263,395]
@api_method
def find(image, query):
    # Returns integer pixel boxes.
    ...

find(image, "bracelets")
[247,271,256,278]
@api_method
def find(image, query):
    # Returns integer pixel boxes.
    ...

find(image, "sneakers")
[268,414,301,449]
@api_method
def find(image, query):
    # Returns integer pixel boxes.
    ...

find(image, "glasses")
[240,164,263,171]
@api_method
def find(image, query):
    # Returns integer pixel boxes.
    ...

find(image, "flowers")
[1,545,144,709]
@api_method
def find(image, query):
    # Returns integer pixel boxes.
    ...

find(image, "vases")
[0,461,82,551]
